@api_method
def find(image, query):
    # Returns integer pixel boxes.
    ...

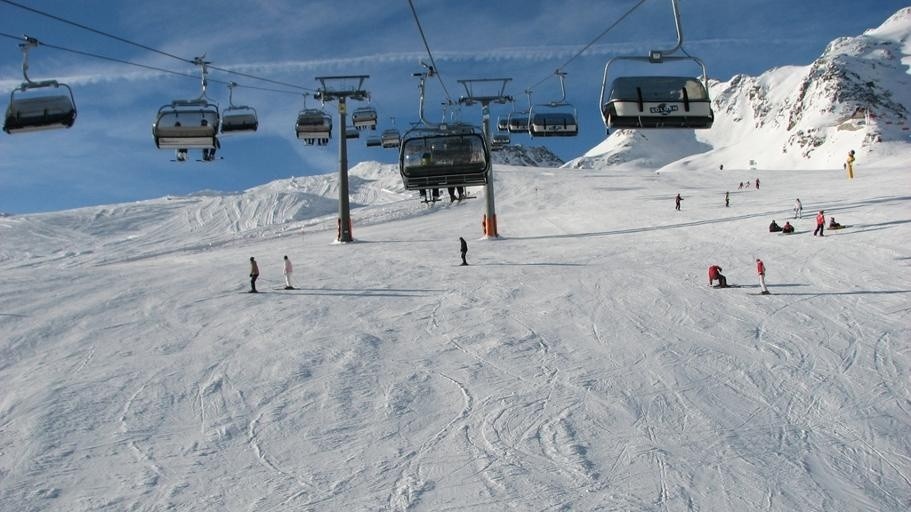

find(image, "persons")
[675,194,684,210]
[283,256,293,289]
[200,119,216,161]
[248,257,259,293]
[447,183,465,202]
[709,265,728,288]
[725,178,760,207]
[848,150,855,180]
[756,258,769,294]
[175,121,187,160]
[419,154,440,200]
[318,138,326,146]
[459,237,468,266]
[308,138,314,145]
[769,199,840,237]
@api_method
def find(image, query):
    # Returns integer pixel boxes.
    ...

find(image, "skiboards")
[450,264,479,267]
[747,293,780,296]
[238,292,268,294]
[712,285,741,288]
[273,288,300,290]
